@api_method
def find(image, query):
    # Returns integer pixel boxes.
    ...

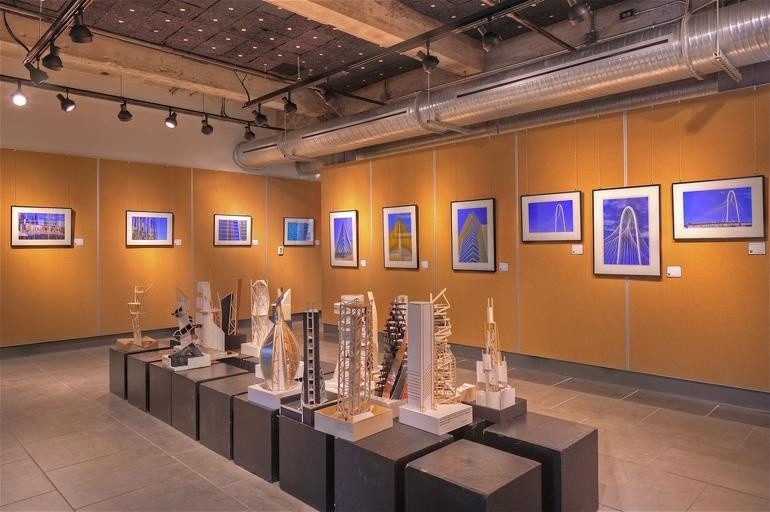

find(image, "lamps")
[252,103,267,126]
[565,0,592,28]
[378,59,396,79]
[201,115,214,136]
[118,101,132,122]
[319,77,337,105]
[416,42,439,73]
[282,91,297,116]
[165,108,176,127]
[477,17,503,54]
[245,123,256,140]
[10,10,93,112]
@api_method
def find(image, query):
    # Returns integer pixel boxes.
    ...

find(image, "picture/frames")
[11,205,74,248]
[329,209,358,269]
[213,214,253,247]
[125,210,173,248]
[591,183,662,279]
[283,217,315,246]
[520,190,583,243]
[382,204,419,270]
[671,175,767,240]
[450,198,497,273]
